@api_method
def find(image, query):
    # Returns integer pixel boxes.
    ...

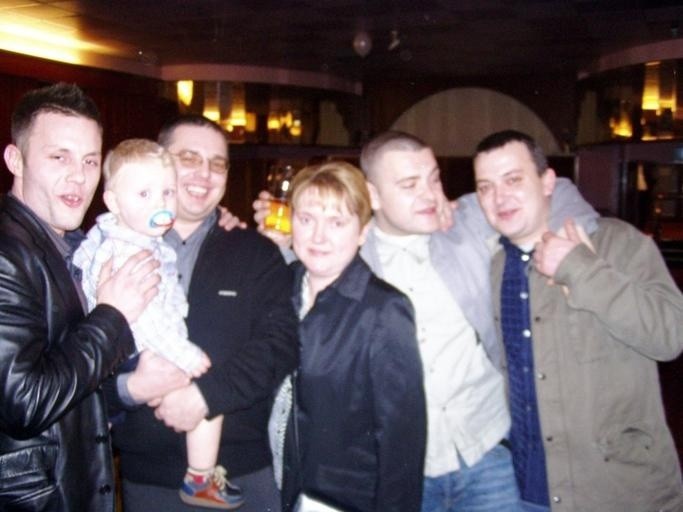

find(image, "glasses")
[170,152,231,174]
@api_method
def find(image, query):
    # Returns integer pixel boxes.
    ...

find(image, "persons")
[0,81,246,512]
[353,129,601,512]
[84,110,301,510]
[69,137,242,508]
[437,129,683,511]
[263,157,425,511]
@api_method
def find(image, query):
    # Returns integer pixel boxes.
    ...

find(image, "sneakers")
[179,468,245,510]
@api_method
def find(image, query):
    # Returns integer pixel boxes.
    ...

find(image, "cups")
[263,163,298,235]
